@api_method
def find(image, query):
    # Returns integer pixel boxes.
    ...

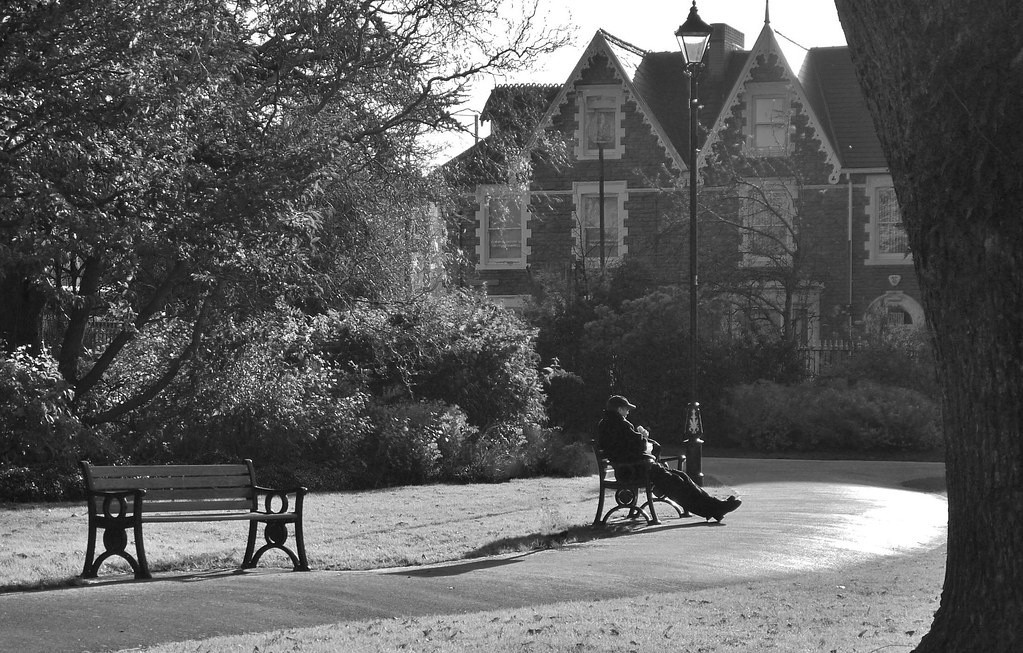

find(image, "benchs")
[77,460,312,582]
[591,437,693,530]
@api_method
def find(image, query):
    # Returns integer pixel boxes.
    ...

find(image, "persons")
[598,395,741,522]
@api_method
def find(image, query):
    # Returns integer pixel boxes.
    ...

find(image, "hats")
[608,395,636,409]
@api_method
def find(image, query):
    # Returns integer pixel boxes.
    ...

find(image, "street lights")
[675,0,714,492]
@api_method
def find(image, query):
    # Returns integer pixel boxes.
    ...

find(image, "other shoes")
[726,495,734,501]
[709,497,742,522]
